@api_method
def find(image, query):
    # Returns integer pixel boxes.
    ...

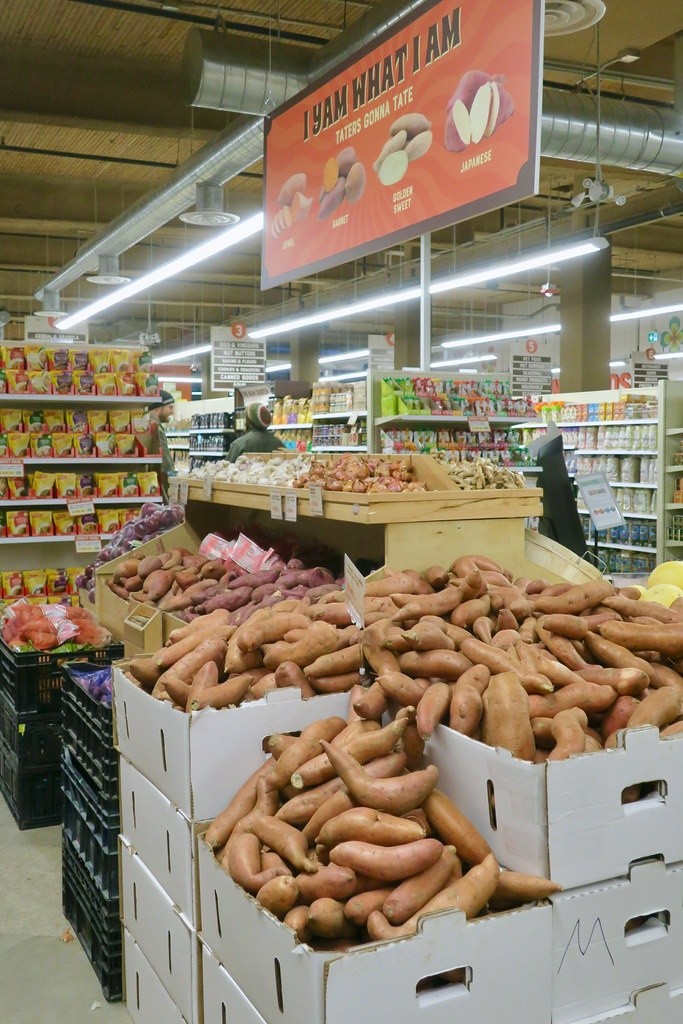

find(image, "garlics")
[177,454,317,488]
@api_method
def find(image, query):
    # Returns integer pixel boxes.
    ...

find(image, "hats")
[148,389,175,411]
[245,403,271,429]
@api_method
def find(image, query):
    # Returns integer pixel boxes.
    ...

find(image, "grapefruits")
[643,585,683,610]
[645,560,683,587]
[628,584,647,600]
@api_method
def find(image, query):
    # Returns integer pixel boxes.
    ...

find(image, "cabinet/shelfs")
[0,393,163,544]
[312,410,367,451]
[164,433,190,449]
[512,379,683,573]
[189,428,235,456]
[366,370,547,471]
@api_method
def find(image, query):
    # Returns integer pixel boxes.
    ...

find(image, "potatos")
[371,113,432,185]
[204,685,562,991]
[116,554,683,809]
[0,601,111,703]
[443,70,514,153]
[105,521,350,626]
[316,147,365,220]
[270,172,312,238]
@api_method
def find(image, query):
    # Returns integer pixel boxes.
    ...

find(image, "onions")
[292,453,429,493]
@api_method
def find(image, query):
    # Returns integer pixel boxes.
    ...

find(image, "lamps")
[653,352,683,360]
[441,303,683,349]
[54,208,264,331]
[551,358,630,374]
[430,354,498,368]
[247,237,610,338]
[265,349,368,381]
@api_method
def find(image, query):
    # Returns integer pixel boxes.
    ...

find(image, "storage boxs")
[0,519,683,1024]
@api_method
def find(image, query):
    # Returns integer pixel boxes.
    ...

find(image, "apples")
[76,502,184,604]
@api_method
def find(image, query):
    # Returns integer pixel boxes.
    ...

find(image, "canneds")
[312,423,351,445]
[329,393,353,413]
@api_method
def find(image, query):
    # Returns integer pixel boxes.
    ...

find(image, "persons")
[150,390,176,492]
[226,402,285,464]
